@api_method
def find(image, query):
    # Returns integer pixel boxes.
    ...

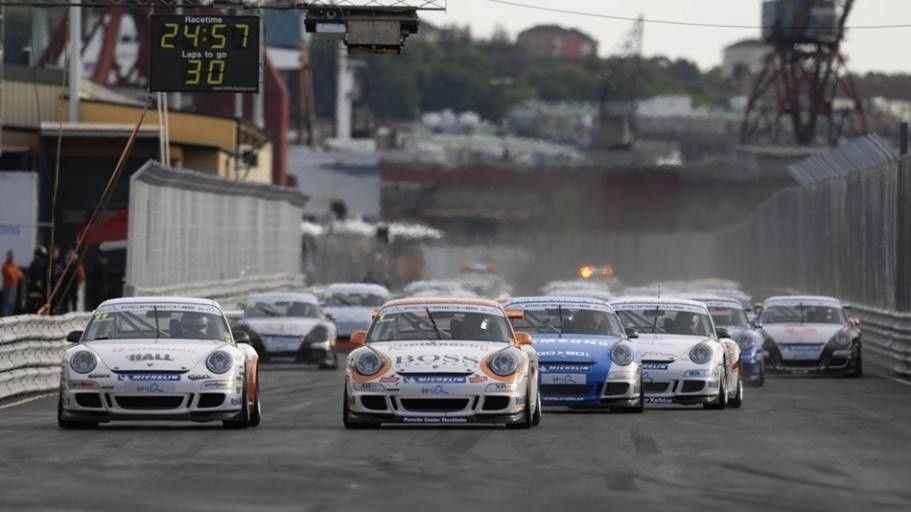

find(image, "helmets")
[34,245,47,258]
